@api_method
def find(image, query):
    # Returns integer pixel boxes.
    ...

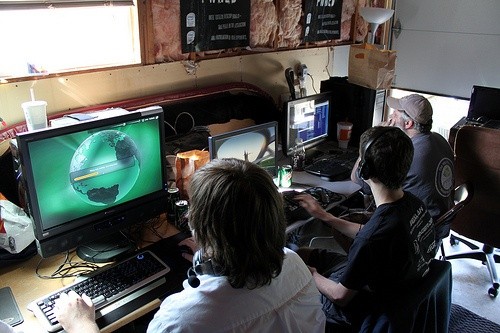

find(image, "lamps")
[359,6,394,44]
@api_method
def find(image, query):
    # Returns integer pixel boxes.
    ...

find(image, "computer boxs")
[321,81,387,142]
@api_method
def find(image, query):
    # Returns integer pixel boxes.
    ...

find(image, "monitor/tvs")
[284,91,331,162]
[466,85,500,124]
[14,105,169,264]
[208,120,279,180]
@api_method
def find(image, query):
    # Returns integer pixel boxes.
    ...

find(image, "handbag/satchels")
[348,43,397,90]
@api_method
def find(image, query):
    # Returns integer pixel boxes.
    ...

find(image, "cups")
[336,122,353,143]
[22,101,47,131]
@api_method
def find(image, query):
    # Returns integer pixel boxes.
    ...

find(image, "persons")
[54,151,326,333]
[293,124,437,333]
[351,94,455,237]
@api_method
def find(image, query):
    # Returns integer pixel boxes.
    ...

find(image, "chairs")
[439,124,500,298]
[352,259,453,333]
[432,182,469,256]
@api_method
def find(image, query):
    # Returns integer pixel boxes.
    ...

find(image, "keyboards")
[29,249,171,333]
[281,190,317,224]
[302,158,355,177]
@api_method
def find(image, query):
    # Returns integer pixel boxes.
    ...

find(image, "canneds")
[166,187,189,231]
[276,165,292,187]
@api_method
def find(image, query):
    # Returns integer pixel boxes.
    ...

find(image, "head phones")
[194,249,230,276]
[357,132,385,179]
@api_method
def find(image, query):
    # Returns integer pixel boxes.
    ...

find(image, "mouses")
[178,245,194,256]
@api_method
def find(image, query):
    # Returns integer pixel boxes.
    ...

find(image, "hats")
[387,94,432,125]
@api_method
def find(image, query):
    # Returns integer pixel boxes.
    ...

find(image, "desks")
[273,171,361,232]
[0,215,183,333]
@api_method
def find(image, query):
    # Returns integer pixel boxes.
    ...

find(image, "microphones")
[187,266,201,289]
